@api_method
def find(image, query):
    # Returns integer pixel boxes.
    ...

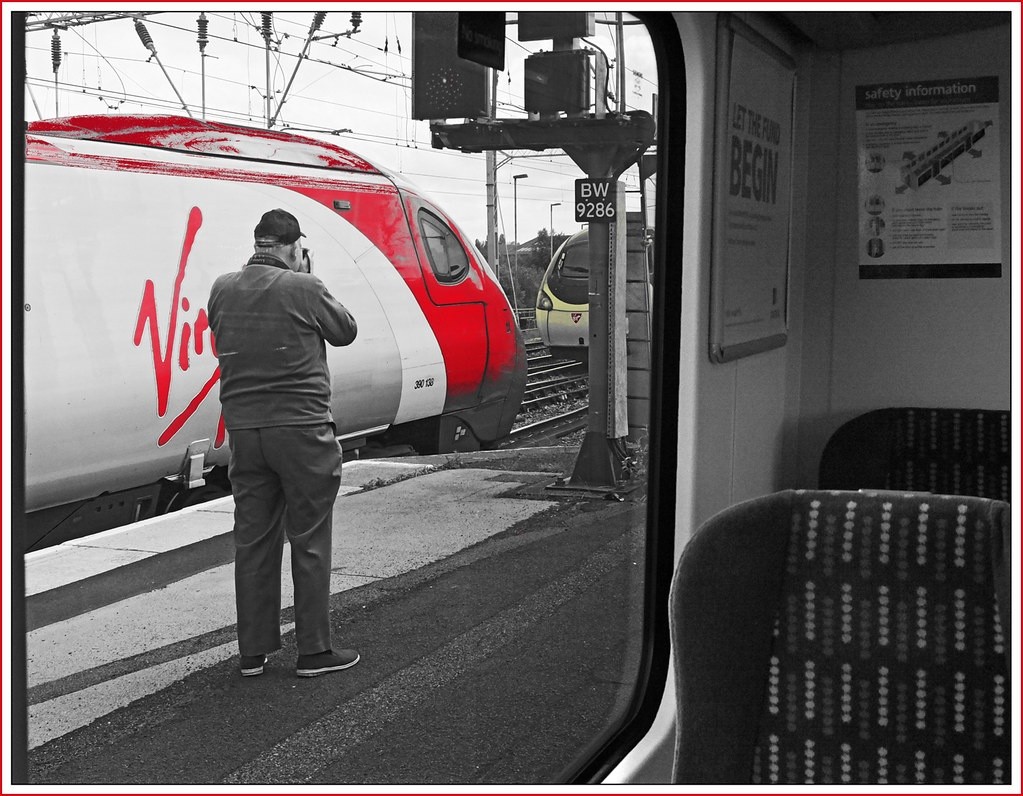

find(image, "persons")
[207,208,362,677]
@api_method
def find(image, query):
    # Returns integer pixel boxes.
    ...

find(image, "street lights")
[549,202,561,263]
[511,173,529,282]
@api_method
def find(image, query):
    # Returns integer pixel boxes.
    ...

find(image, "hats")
[254,209,306,247]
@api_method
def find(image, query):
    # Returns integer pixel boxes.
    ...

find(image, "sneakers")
[296,649,360,676]
[239,655,267,676]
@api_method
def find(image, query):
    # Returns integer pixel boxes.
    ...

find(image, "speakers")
[409,12,492,122]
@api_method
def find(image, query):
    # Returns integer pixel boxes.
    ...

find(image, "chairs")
[666,488,1012,786]
[818,407,1011,507]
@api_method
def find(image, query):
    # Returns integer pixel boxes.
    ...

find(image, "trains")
[538,230,659,359]
[22,112,526,515]
